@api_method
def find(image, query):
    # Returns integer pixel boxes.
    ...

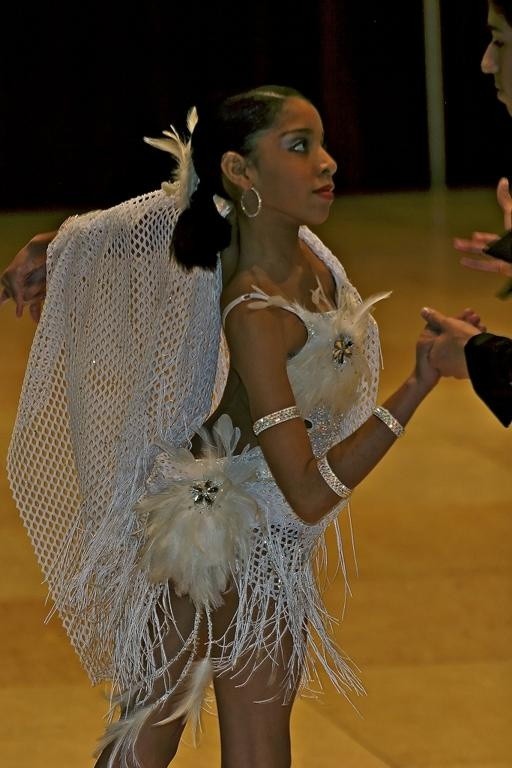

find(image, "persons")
[0,87,488,768]
[419,0,511,428]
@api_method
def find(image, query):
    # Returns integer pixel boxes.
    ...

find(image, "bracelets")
[372,402,407,441]
